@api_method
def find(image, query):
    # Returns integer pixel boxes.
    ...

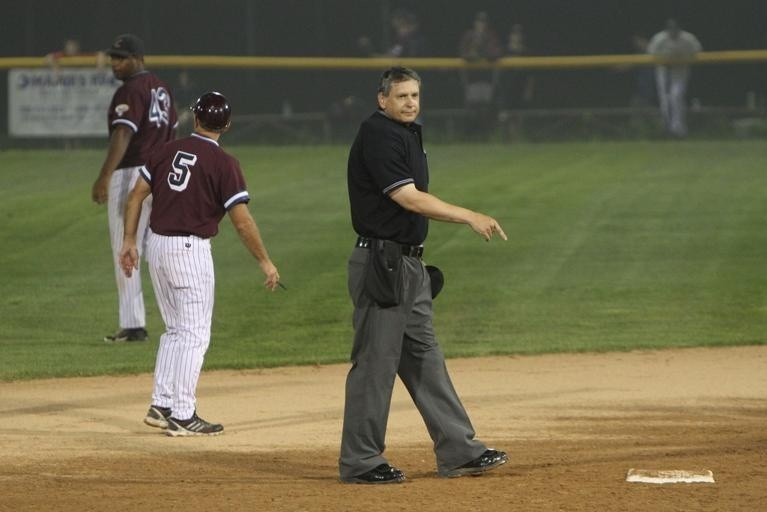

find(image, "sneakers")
[447,449,509,478]
[143,405,224,437]
[103,330,149,343]
[342,464,406,484]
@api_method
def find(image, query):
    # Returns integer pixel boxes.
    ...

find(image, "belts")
[355,235,424,258]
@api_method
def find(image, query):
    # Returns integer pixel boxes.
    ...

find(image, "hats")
[105,35,143,59]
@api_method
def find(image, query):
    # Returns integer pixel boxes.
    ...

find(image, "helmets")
[189,91,231,129]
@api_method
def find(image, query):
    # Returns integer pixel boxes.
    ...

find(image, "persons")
[645,16,703,138]
[120,92,280,437]
[357,13,446,60]
[502,24,536,108]
[91,34,181,344]
[452,12,502,105]
[338,66,508,485]
[44,40,105,83]
[616,33,659,132]
